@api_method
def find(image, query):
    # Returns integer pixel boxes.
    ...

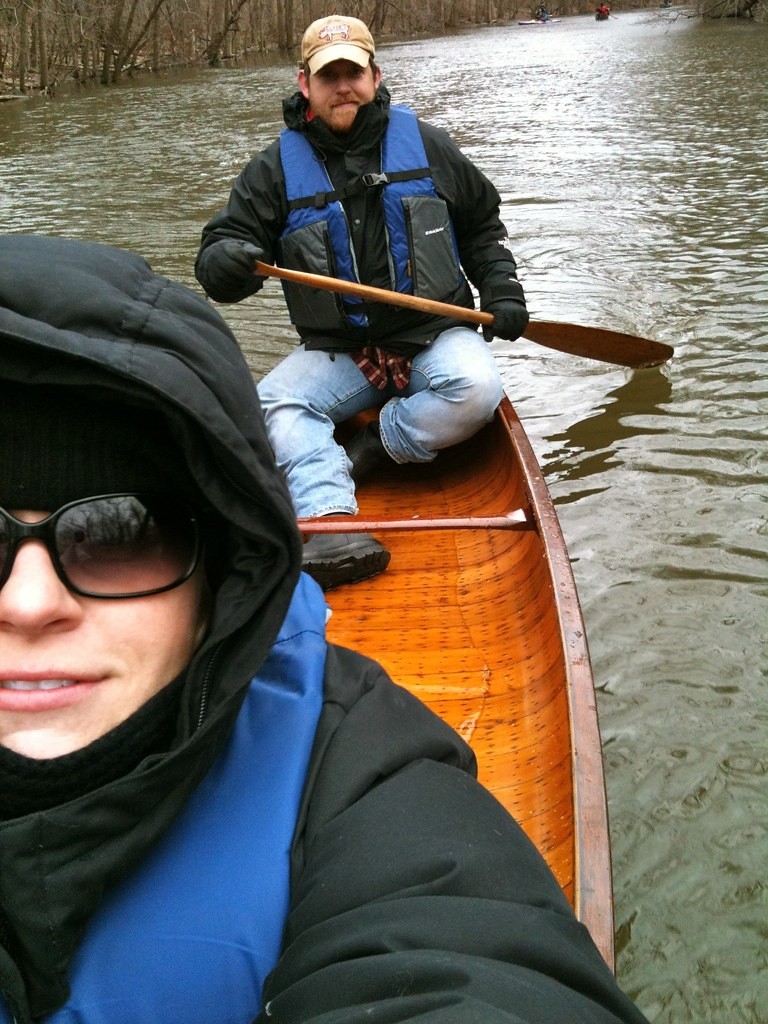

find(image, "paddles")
[248,257,672,371]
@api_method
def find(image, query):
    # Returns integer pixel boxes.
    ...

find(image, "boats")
[595,12,609,21]
[517,19,563,25]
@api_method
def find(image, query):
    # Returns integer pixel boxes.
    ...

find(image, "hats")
[0,375,228,531]
[297,16,376,76]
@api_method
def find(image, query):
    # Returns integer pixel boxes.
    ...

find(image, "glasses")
[0,492,228,600]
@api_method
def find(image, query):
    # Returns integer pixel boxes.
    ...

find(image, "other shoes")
[344,420,395,484]
[299,534,391,593]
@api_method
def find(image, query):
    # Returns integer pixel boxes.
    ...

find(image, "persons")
[193,14,530,592]
[535,6,548,22]
[0,232,652,1023]
[594,3,610,21]
[664,0,669,8]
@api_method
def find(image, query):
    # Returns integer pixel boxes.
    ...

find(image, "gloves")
[481,299,529,343]
[197,239,264,293]
